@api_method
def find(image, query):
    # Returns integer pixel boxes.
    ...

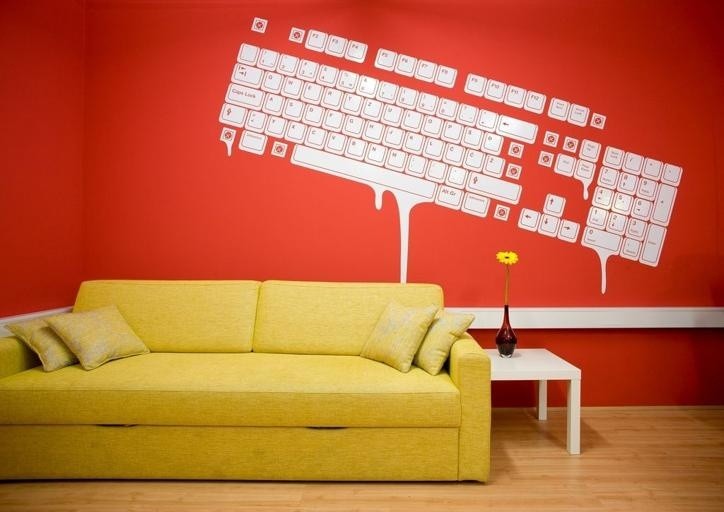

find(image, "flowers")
[495,249,519,304]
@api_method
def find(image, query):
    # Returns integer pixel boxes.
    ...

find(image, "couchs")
[0,279,491,484]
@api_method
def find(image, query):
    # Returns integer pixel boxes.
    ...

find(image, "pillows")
[414,309,476,376]
[360,304,439,374]
[43,304,151,371]
[3,318,78,372]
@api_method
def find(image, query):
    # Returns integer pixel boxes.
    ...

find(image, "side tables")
[481,347,582,455]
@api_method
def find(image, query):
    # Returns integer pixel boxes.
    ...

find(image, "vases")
[495,305,517,359]
